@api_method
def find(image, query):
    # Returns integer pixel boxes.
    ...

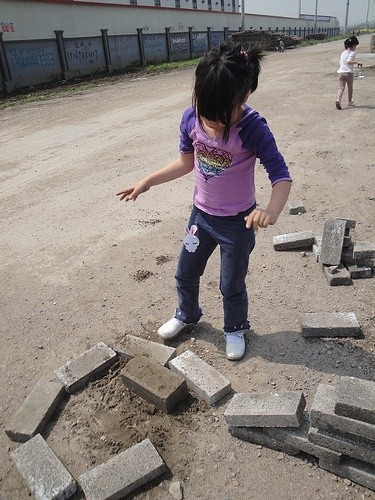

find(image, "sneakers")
[335,100,342,109]
[158,316,186,339]
[348,102,353,106]
[225,331,245,360]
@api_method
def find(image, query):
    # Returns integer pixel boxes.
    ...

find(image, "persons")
[335,35,363,110]
[115,38,293,361]
